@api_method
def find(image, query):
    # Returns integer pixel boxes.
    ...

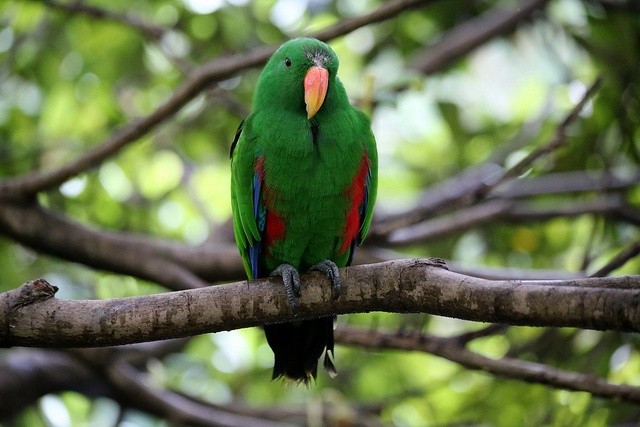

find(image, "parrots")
[228,34,378,391]
